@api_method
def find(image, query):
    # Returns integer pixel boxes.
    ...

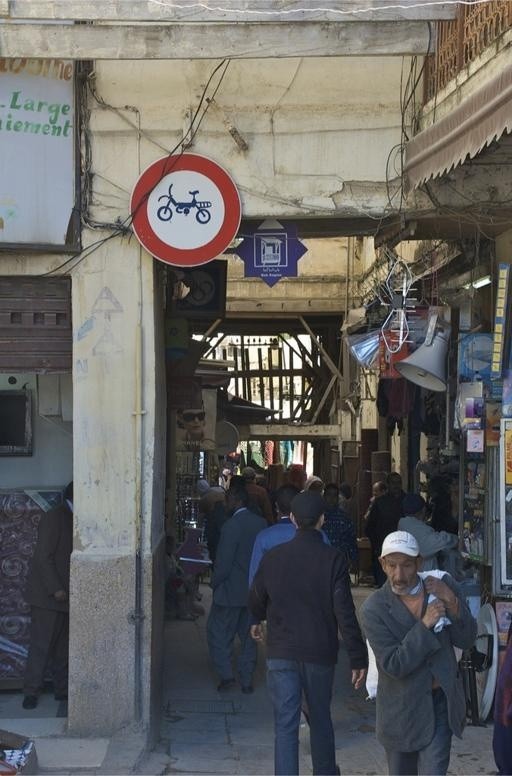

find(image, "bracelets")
[446,595,459,608]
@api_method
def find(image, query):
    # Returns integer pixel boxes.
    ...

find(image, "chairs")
[2,481,73,692]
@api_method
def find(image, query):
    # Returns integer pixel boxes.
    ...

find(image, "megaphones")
[393,313,452,392]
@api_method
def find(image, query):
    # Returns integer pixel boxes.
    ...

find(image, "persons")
[359,530,478,775]
[195,462,461,694]
[483,619,512,775]
[22,479,74,711]
[246,489,368,776]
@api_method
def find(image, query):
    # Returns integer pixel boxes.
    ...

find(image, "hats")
[242,467,255,478]
[288,464,307,481]
[401,494,425,514]
[380,530,420,557]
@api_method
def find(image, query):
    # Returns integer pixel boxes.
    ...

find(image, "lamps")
[458,273,492,294]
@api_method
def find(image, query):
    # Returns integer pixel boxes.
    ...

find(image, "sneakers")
[291,494,324,518]
[218,678,253,693]
[22,696,37,709]
